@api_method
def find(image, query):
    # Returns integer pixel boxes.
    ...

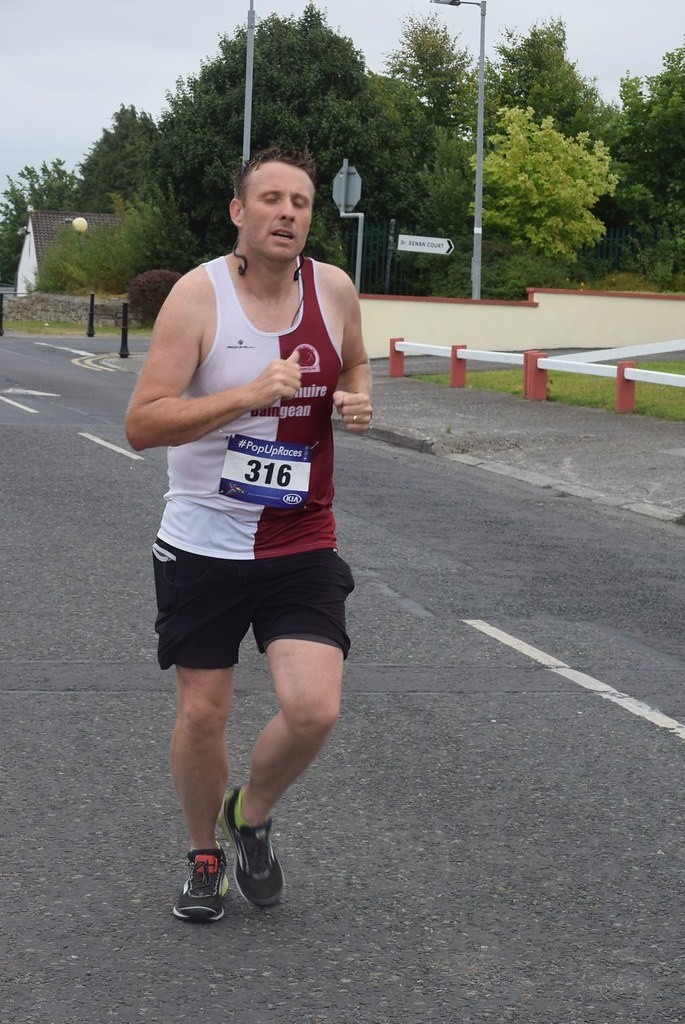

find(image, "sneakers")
[218,785,284,907]
[173,840,229,921]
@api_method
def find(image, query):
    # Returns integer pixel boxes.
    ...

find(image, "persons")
[125,146,374,922]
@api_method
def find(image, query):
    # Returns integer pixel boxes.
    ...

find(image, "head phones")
[233,240,305,281]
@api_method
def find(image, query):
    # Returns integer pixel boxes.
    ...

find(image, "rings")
[353,415,356,424]
